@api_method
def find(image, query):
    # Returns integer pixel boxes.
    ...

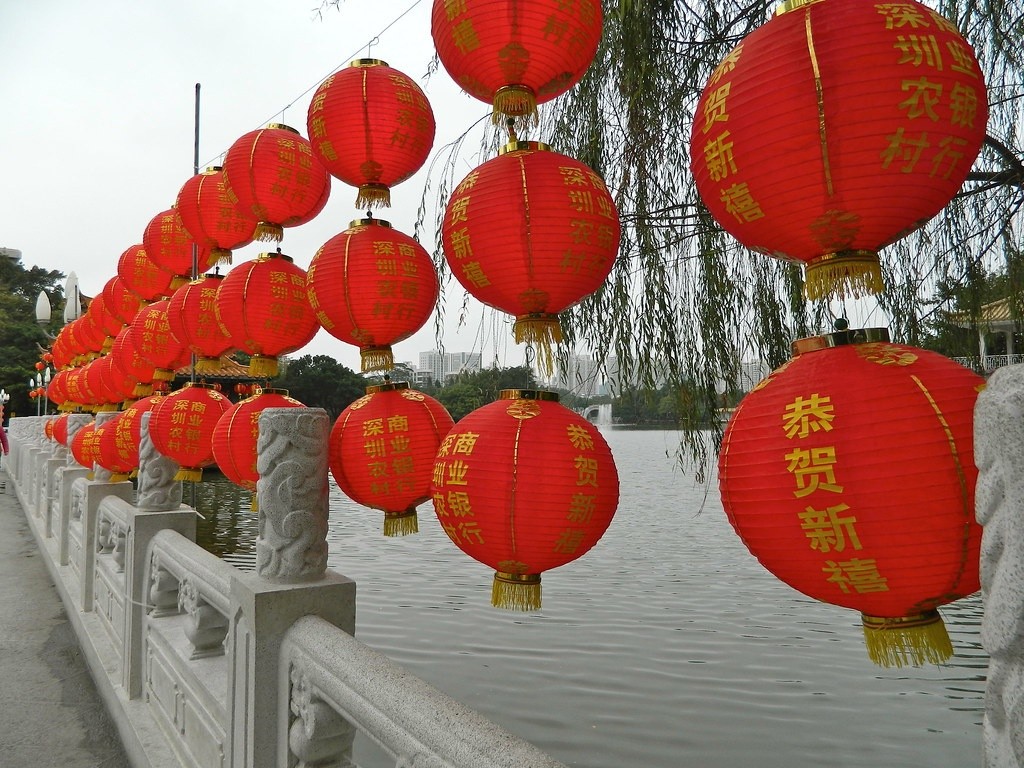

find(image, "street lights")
[35,272,81,342]
[0,389,10,426]
[44,367,50,415]
[30,373,42,417]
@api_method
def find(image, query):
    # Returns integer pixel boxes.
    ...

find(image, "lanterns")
[689,0,990,303]
[716,318,995,669]
[304,210,440,375]
[441,140,621,377]
[147,375,235,483]
[211,377,310,513]
[306,37,436,210]
[182,378,271,400]
[142,205,219,291]
[165,266,235,375]
[430,388,620,612]
[328,374,456,538]
[430,0,604,129]
[174,154,262,266]
[221,109,332,244]
[29,244,194,485]
[213,247,321,377]
